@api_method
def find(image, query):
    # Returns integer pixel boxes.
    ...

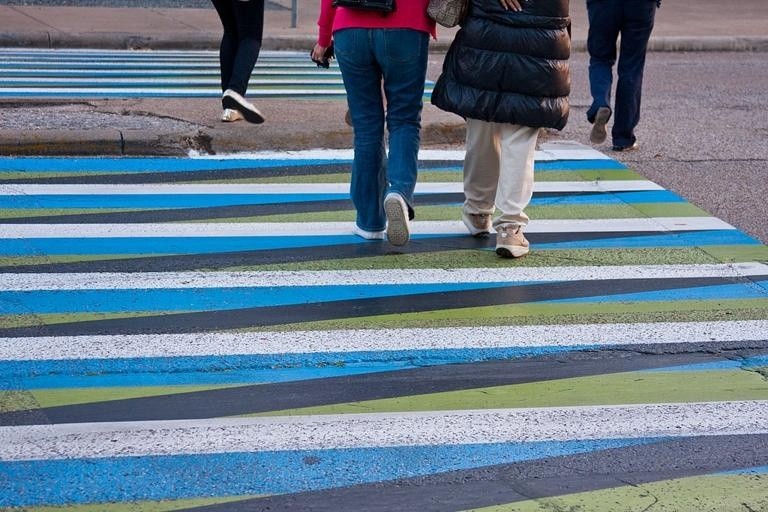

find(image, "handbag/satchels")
[426,0,468,28]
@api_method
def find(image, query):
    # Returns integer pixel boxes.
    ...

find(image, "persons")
[205,1,270,125]
[427,1,573,258]
[309,1,437,254]
[581,0,663,154]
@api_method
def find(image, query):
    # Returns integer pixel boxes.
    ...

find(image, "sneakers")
[494,224,530,258]
[351,220,385,241]
[613,142,640,152]
[382,191,411,247]
[589,106,611,144]
[220,87,265,125]
[459,208,491,239]
[221,108,246,123]
